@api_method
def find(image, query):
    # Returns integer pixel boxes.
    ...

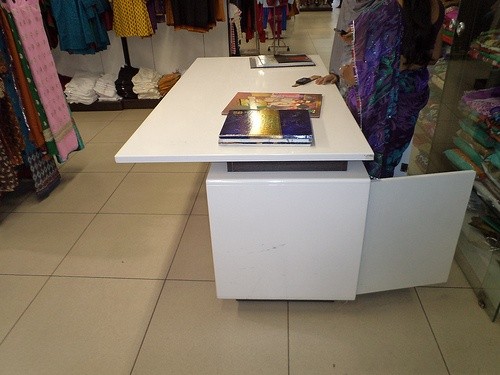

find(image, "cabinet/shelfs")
[114,54,475,300]
[328,0,500,324]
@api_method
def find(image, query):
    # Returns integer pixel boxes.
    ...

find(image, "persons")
[315,0,444,179]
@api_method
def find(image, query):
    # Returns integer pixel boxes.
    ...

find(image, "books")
[218,107,313,147]
